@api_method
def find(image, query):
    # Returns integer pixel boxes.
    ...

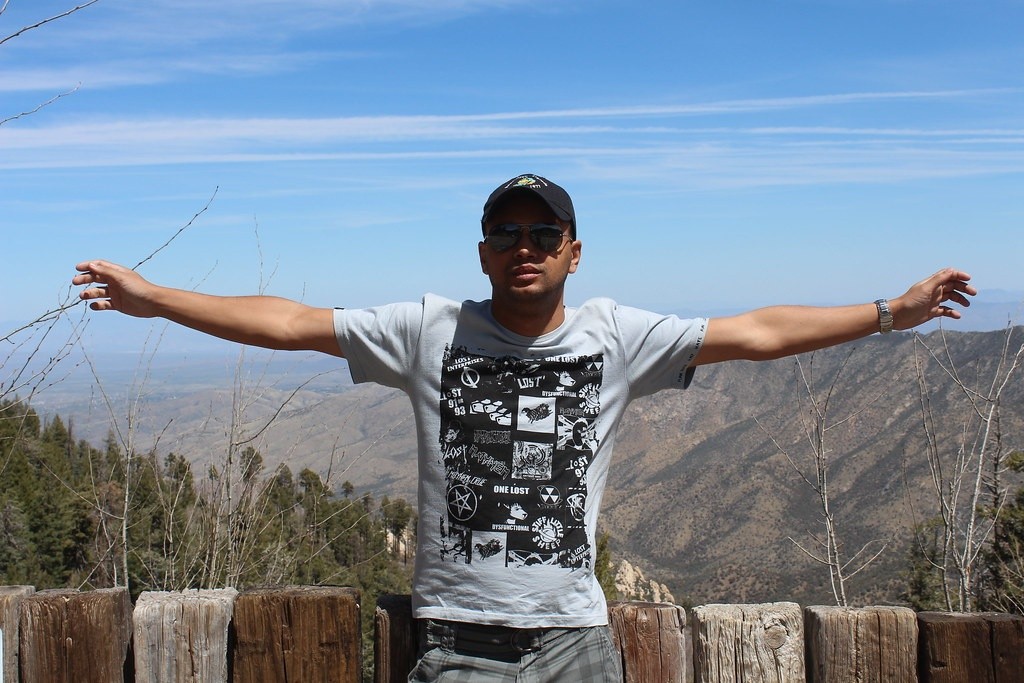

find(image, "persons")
[71,175,977,683]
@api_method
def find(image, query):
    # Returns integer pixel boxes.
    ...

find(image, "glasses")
[484,223,573,253]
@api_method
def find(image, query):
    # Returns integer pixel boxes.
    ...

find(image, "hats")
[481,174,576,241]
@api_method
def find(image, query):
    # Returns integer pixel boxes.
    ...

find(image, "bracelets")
[873,297,893,335]
